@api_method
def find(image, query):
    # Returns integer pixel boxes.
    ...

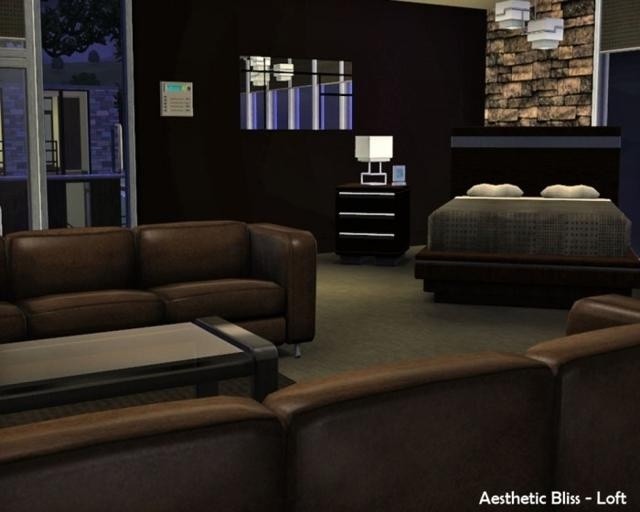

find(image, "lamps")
[494,0,564,51]
[249,55,295,87]
[355,134,393,186]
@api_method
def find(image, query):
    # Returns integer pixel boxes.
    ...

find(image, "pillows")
[540,183,601,198]
[467,183,525,197]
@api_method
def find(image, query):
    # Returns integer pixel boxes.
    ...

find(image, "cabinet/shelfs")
[335,181,412,267]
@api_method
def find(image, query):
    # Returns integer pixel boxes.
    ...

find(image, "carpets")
[0,368,300,430]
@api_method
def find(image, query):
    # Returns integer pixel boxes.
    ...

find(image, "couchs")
[0,220,317,358]
[0,295,640,512]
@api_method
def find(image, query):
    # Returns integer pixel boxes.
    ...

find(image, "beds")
[414,127,640,308]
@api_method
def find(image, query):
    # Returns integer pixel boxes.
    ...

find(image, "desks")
[0,314,279,401]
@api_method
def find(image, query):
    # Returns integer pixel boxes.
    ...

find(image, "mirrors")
[237,54,354,131]
